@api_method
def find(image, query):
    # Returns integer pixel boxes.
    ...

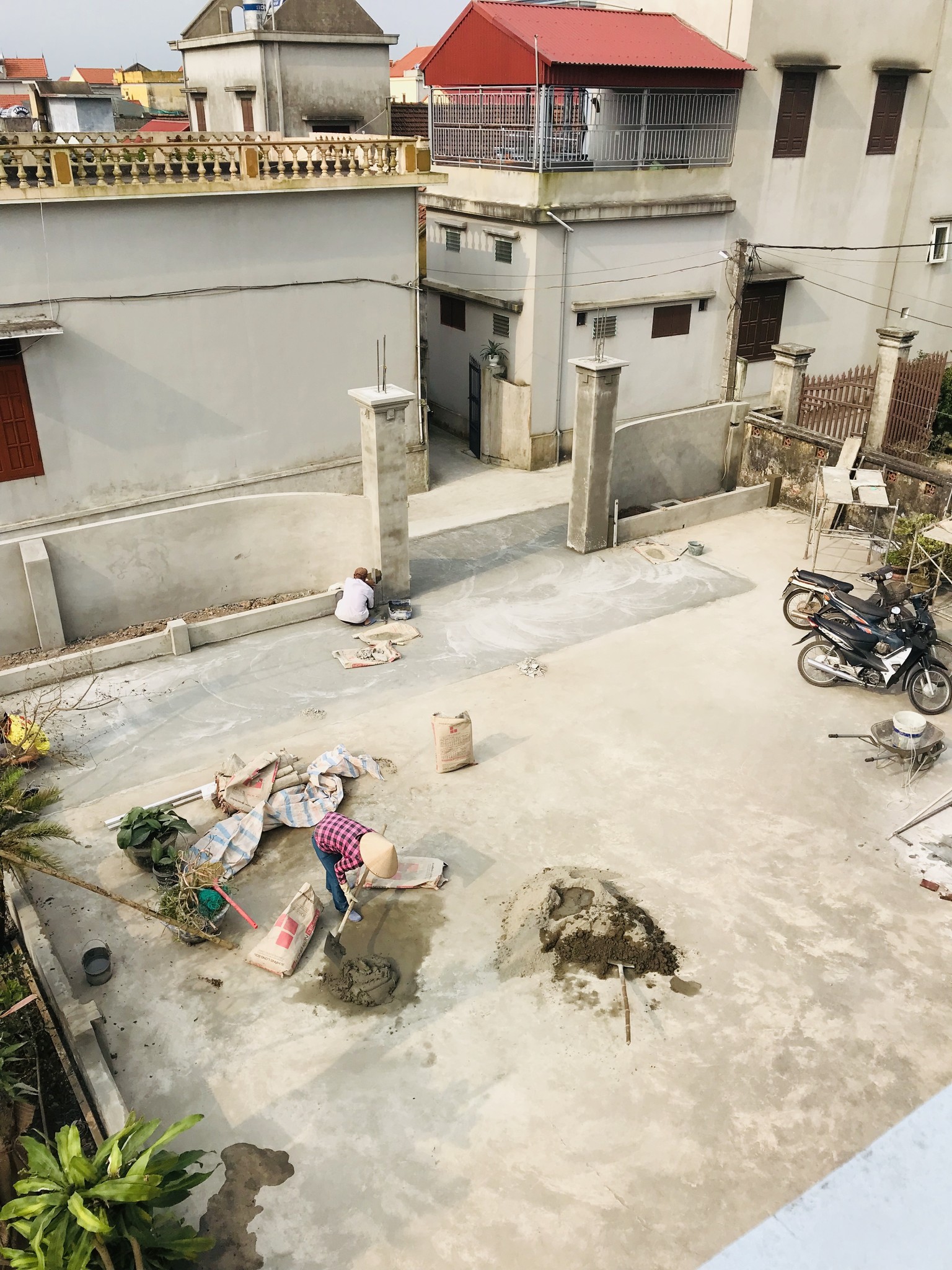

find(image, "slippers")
[363,617,376,627]
[24,767,37,774]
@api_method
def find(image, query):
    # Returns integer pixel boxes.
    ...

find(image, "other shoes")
[348,882,354,889]
[342,910,362,922]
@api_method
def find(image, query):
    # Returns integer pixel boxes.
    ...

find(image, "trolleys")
[828,718,948,771]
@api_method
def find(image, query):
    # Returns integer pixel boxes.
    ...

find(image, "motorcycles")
[778,562,914,630]
[817,577,952,687]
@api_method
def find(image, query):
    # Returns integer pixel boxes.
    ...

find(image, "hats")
[355,568,368,578]
[0,707,6,721]
[359,832,398,879]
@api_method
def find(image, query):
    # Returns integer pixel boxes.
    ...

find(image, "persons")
[312,811,399,922]
[335,568,377,627]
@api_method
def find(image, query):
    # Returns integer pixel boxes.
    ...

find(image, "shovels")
[324,823,388,968]
[607,957,635,1045]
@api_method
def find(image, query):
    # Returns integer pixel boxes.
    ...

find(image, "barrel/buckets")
[891,711,927,749]
[80,939,112,985]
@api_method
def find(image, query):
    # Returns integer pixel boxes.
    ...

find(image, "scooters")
[792,606,952,715]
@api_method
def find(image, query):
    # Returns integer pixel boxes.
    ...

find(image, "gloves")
[339,881,358,905]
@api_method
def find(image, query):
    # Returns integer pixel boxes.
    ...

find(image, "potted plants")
[878,503,950,606]
[479,338,510,368]
[145,832,240,946]
[115,796,196,875]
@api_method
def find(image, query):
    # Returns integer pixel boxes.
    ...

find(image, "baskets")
[884,581,912,603]
